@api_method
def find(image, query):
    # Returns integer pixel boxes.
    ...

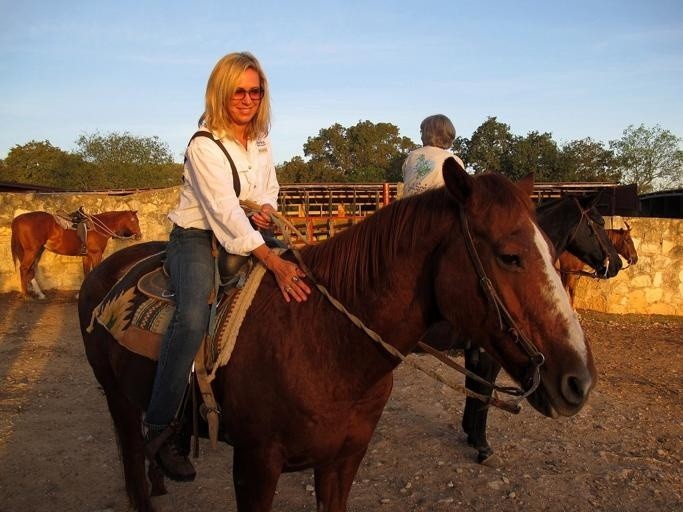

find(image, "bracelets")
[263,251,274,266]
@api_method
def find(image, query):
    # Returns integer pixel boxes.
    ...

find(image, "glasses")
[229,89,264,99]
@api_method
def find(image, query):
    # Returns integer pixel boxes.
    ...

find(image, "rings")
[290,275,300,282]
[284,285,291,291]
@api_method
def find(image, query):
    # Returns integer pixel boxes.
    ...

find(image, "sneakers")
[144,429,195,482]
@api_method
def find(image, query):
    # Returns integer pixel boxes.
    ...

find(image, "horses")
[460,193,621,468]
[77,156,599,512]
[10,210,142,301]
[553,222,638,305]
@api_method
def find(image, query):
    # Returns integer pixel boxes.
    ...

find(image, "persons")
[399,114,464,202]
[143,52,313,483]
